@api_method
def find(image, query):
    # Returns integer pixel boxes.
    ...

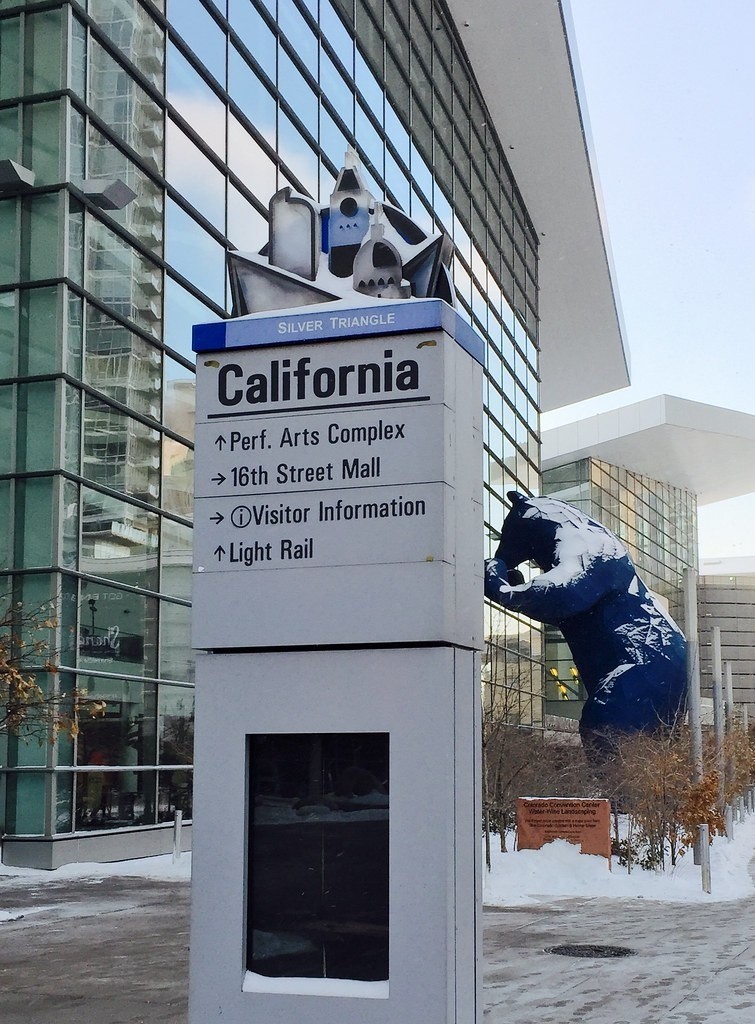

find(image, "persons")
[91,738,138,819]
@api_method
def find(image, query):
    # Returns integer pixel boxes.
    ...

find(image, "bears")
[484,489,691,815]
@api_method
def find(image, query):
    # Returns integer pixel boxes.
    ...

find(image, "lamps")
[0,158,36,189]
[83,178,138,208]
